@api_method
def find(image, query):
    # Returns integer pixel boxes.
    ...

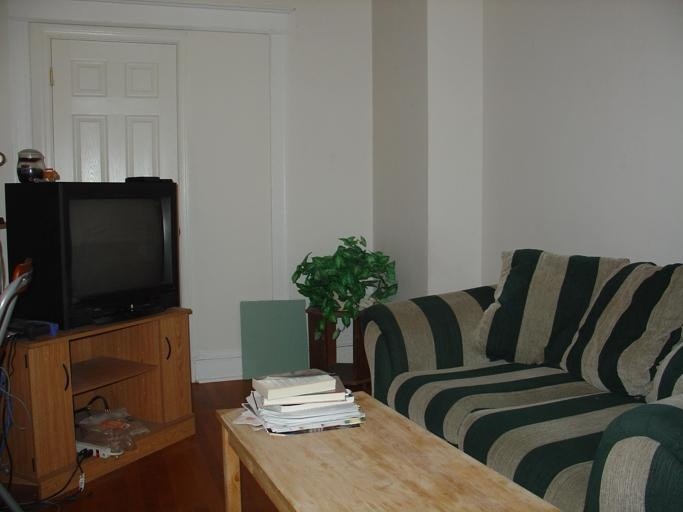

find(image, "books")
[232,368,366,435]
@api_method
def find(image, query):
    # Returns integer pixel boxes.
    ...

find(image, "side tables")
[305,304,373,394]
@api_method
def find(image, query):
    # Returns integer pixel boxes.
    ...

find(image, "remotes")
[125,176,161,183]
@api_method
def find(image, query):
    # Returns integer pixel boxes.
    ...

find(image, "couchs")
[347,283,683,510]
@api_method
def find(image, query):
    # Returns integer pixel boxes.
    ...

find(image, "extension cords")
[76,441,111,459]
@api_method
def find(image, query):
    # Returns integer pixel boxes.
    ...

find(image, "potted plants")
[290,234,400,344]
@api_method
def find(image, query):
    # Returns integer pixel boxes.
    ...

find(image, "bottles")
[16,146,44,182]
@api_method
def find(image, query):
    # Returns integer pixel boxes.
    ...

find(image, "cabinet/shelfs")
[0,304,197,506]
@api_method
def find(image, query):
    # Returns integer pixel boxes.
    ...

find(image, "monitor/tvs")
[5,180,180,330]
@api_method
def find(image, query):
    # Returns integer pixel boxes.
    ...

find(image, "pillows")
[473,248,683,403]
